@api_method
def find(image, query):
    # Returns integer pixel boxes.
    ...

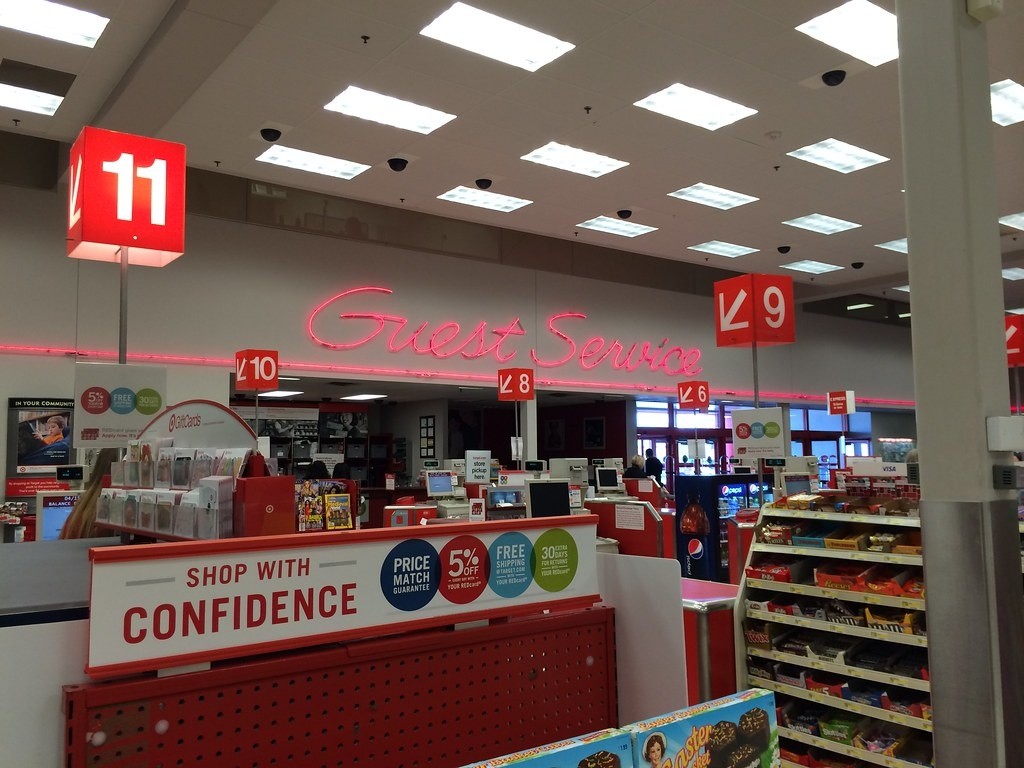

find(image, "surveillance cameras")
[617,210,631,219]
[822,70,846,86]
[260,128,281,142]
[475,179,492,190]
[777,246,791,253]
[387,158,408,172]
[852,262,864,269]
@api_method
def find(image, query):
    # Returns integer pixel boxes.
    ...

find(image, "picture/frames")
[325,494,352,529]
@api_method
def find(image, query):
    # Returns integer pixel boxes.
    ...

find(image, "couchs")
[32,426,70,465]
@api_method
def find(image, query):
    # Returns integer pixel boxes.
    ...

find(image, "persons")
[333,463,351,479]
[32,416,65,446]
[273,419,293,436]
[339,412,361,434]
[646,449,663,482]
[302,460,331,480]
[301,480,315,496]
[305,499,322,515]
[2,503,27,516]
[622,455,647,478]
[58,447,127,541]
[330,485,339,494]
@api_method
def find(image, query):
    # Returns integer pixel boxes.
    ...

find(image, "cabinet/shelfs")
[93,474,237,540]
[732,489,935,768]
[393,441,413,476]
[270,436,388,490]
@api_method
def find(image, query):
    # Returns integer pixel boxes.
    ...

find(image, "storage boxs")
[383,505,437,528]
[271,442,387,480]
[742,494,935,768]
[456,687,781,768]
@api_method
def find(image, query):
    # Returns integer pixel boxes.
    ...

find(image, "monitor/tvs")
[35,490,88,541]
[595,467,620,490]
[524,478,571,519]
[734,466,751,473]
[426,470,455,497]
[780,472,811,496]
